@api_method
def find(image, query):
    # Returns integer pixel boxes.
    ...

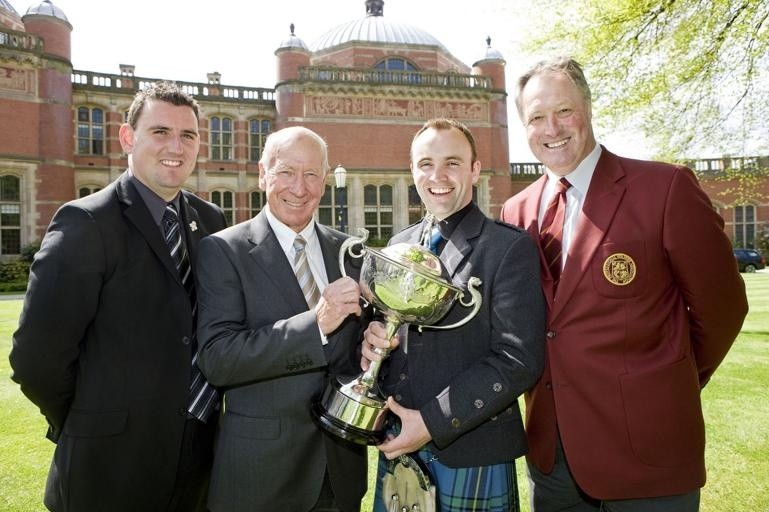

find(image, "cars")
[733,249,766,273]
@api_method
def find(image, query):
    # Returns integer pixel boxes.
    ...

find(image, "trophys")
[310,225,483,447]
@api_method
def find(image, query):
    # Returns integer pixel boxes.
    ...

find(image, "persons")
[8,84,229,510]
[194,126,377,512]
[361,116,545,512]
[501,57,748,512]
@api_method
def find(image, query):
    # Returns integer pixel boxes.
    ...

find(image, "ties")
[164,203,219,423]
[424,227,441,255]
[539,178,572,298]
[292,234,321,311]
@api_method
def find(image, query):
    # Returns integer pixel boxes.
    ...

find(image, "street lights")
[333,164,347,232]
